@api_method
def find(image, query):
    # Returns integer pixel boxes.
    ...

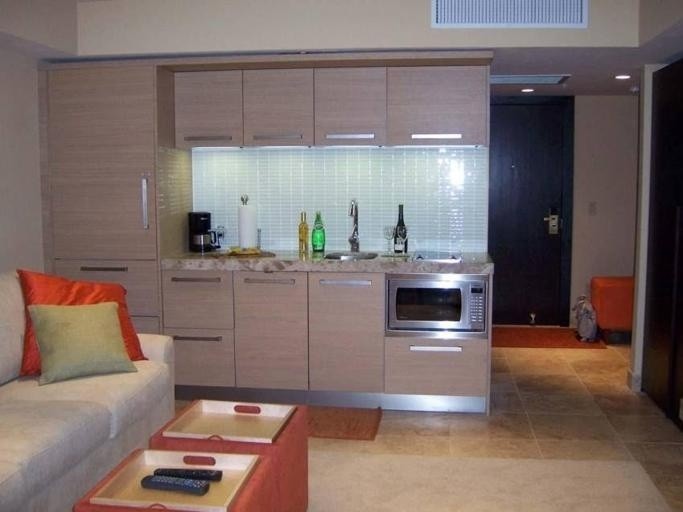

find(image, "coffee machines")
[186,211,216,252]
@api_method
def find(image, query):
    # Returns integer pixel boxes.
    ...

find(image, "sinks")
[324,251,379,261]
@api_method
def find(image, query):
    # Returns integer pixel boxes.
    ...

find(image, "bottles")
[297,212,308,253]
[255,228,262,249]
[393,205,407,253]
[213,228,223,248]
[311,212,324,251]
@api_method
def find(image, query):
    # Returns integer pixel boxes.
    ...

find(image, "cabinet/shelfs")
[379,337,489,398]
[310,66,386,149]
[241,66,315,151]
[38,54,192,260]
[228,271,384,395]
[42,261,162,336]
[158,270,236,391]
[382,67,490,151]
[170,68,244,149]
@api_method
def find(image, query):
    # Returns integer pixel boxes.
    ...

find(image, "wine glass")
[383,225,395,255]
[396,226,408,255]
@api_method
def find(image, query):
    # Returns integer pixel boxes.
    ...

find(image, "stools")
[589,273,633,346]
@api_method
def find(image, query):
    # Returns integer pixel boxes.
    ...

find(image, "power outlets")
[214,229,225,240]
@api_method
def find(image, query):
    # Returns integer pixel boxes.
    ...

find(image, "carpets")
[491,325,608,350]
[304,448,673,512]
[308,405,383,442]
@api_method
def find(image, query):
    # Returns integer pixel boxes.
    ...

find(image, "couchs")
[1,265,175,512]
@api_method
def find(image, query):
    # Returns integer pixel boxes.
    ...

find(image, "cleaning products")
[311,211,326,252]
[312,251,325,261]
[298,212,310,253]
[299,252,309,261]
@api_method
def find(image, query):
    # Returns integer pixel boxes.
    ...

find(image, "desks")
[71,446,274,512]
[147,396,311,512]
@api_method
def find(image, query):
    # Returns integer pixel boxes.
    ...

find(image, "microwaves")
[385,277,489,341]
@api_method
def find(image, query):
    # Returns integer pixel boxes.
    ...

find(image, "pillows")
[18,269,145,378]
[26,299,137,386]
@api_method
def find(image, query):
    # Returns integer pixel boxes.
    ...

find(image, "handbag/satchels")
[572,299,598,340]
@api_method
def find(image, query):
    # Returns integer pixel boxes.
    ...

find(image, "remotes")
[141,475,213,496]
[155,468,222,481]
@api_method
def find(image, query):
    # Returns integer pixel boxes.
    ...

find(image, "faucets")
[348,199,359,252]
